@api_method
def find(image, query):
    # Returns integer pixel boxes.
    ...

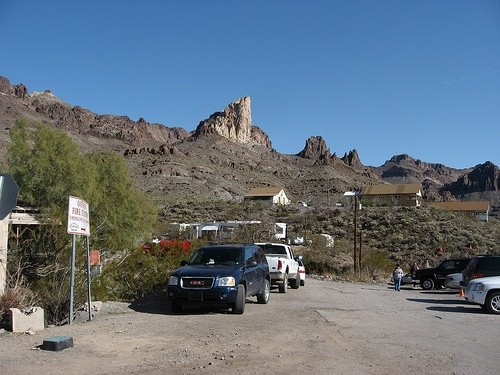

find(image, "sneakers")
[395,289,397,291]
[398,290,400,291]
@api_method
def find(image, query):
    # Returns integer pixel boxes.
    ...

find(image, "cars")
[446,272,467,289]
[465,276,500,314]
[298,261,306,286]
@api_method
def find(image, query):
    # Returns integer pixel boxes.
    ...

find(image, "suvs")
[168,242,271,313]
[415,259,469,290]
[459,256,500,289]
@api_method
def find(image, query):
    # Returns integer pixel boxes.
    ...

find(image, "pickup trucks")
[252,243,304,293]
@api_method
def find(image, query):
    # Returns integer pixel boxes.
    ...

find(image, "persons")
[410,263,419,287]
[392,264,404,291]
